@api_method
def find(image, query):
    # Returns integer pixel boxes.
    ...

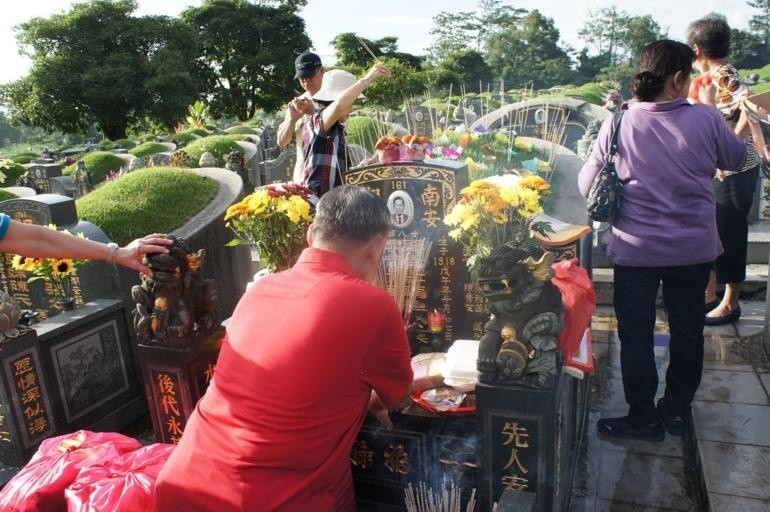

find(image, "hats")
[293,52,322,80]
[313,69,365,102]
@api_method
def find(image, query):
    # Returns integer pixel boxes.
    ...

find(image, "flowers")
[10,222,103,308]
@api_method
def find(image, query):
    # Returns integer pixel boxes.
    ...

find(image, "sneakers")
[657,398,687,435]
[597,415,664,441]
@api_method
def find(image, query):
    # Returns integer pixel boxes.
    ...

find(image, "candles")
[427,307,447,333]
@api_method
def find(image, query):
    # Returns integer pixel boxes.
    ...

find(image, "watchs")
[104,242,119,262]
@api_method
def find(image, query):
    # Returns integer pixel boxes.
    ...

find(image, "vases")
[55,294,77,314]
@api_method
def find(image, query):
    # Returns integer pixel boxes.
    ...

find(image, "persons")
[36,171,41,178]
[577,40,747,442]
[687,19,761,325]
[596,427,690,511]
[535,111,544,123]
[604,89,620,111]
[0,209,175,277]
[278,54,348,187]
[301,62,391,197]
[416,113,422,120]
[391,196,408,223]
[153,185,416,511]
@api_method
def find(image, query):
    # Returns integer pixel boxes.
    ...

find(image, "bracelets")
[362,75,369,86]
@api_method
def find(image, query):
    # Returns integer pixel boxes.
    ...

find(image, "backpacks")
[585,162,620,222]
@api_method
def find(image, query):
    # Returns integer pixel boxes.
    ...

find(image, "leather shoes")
[704,297,741,325]
